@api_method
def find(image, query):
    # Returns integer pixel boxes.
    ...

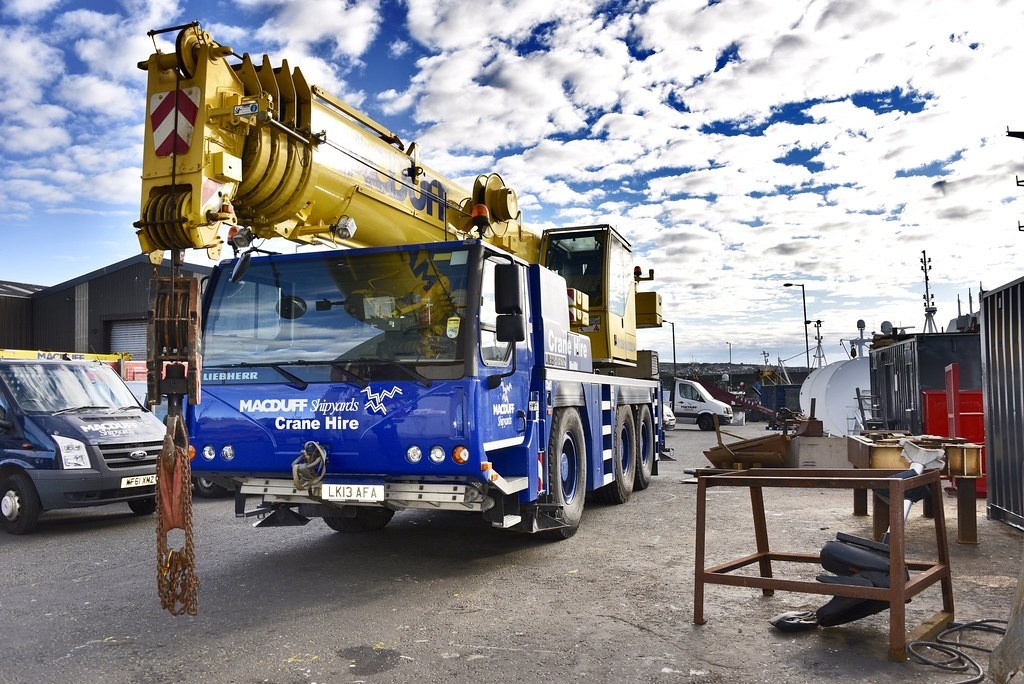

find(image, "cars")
[126,381,228,499]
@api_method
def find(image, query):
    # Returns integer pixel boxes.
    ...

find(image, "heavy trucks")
[105,204,668,542]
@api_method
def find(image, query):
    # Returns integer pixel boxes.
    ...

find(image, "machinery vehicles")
[131,17,664,610]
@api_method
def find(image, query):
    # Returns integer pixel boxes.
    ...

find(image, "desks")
[848,434,983,545]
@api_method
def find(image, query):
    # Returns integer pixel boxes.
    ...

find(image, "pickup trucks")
[661,378,734,432]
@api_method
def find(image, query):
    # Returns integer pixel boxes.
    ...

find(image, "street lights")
[661,319,677,378]
[725,342,732,388]
[783,283,811,375]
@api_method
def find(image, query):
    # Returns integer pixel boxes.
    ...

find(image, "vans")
[0,360,169,537]
